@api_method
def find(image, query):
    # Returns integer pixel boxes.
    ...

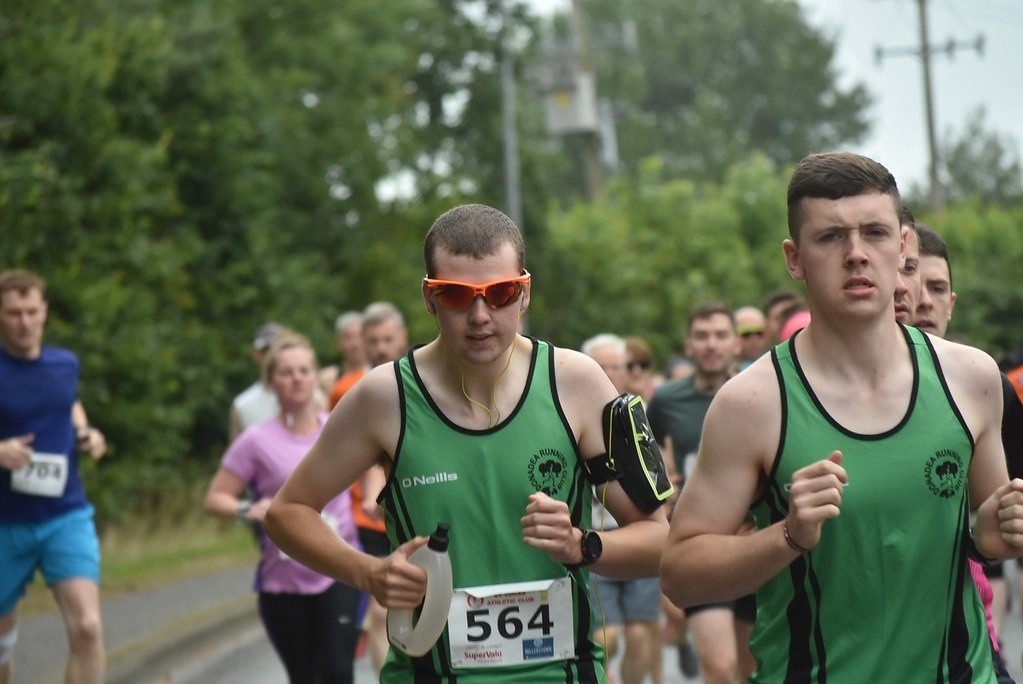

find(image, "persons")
[619,206,1023,684]
[658,149,1023,684]
[327,298,407,671]
[0,267,108,684]
[317,310,375,658]
[261,203,672,684]
[642,301,748,679]
[580,330,658,683]
[229,318,334,535]
[202,334,368,683]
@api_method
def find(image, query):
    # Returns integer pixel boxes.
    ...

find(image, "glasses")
[423,269,531,313]
[627,360,651,371]
[738,327,765,341]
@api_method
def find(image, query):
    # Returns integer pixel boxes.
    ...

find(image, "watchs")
[563,526,603,570]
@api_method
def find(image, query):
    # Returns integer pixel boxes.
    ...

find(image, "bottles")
[387,521,454,657]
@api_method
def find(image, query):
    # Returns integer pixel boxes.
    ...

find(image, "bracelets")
[783,519,814,555]
[238,500,250,520]
